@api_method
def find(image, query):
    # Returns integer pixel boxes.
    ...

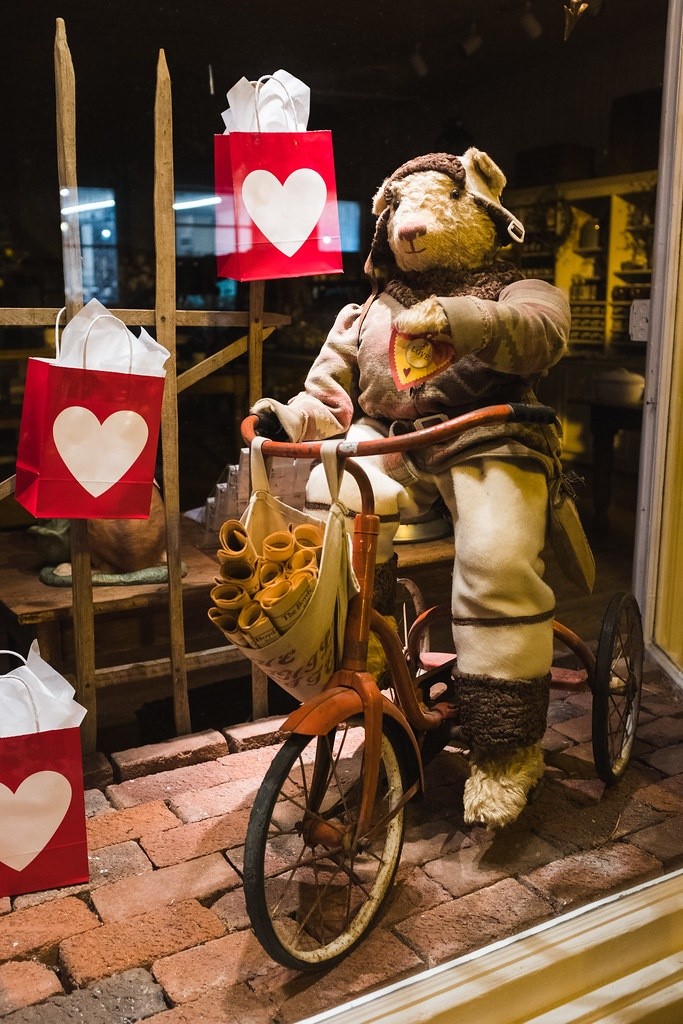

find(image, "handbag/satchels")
[214,75,344,281]
[14,305,165,521]
[0,649,88,898]
[211,433,350,701]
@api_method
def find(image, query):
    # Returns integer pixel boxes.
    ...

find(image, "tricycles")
[237,409,643,975]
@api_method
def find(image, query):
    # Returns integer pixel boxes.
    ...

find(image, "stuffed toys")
[250,146,570,827]
